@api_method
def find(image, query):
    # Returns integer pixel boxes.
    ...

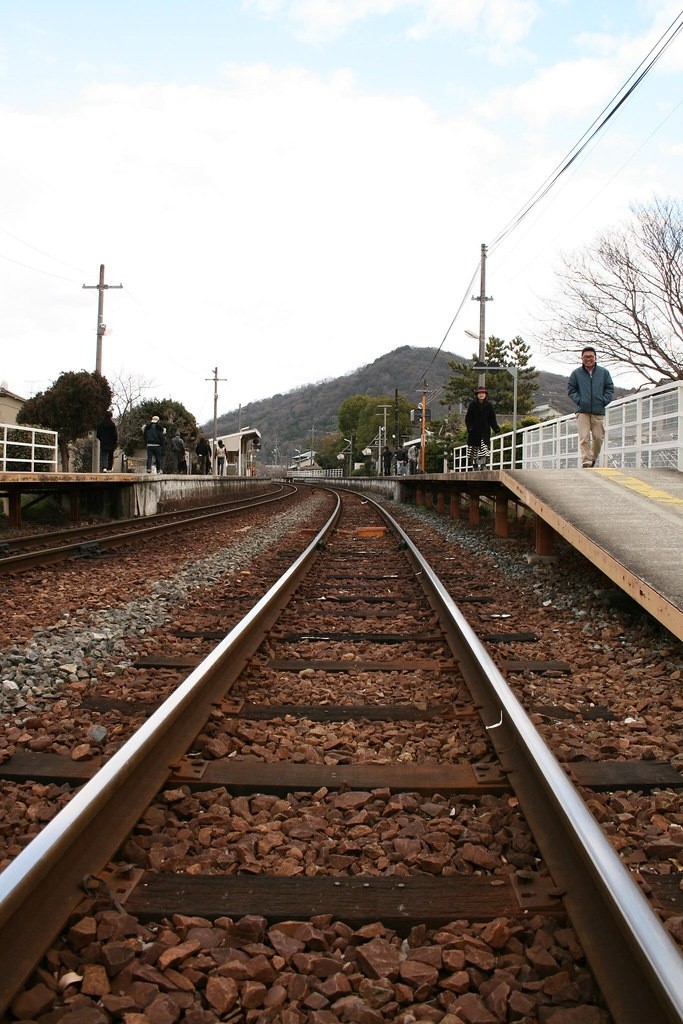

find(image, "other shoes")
[473,464,480,471]
[480,464,488,471]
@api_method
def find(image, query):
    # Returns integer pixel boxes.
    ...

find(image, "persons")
[169,432,185,475]
[214,439,228,477]
[566,346,614,468]
[407,443,418,475]
[380,446,392,476]
[392,445,405,476]
[464,386,501,472]
[142,415,166,475]
[96,411,117,473]
[195,437,212,476]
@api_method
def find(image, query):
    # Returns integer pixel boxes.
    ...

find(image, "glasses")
[583,355,595,359]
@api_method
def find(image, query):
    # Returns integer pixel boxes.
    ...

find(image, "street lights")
[344,438,352,477]
[92,322,107,473]
[295,448,300,471]
[464,328,485,387]
[212,392,219,475]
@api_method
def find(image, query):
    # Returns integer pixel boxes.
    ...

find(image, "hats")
[151,415,160,424]
[474,387,488,395]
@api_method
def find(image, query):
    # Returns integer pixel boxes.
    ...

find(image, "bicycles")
[526,434,678,469]
